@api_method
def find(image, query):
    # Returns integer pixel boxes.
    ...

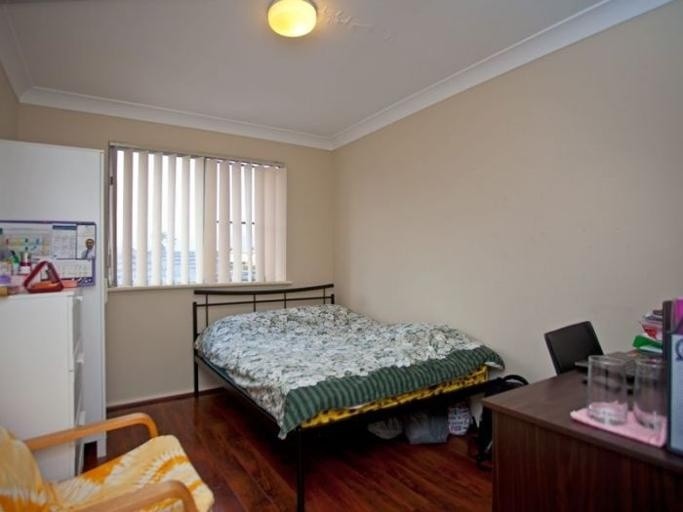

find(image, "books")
[638,307,665,345]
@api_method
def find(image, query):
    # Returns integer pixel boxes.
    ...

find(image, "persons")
[81,237,95,259]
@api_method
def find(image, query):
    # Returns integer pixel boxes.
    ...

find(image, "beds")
[190,282,507,511]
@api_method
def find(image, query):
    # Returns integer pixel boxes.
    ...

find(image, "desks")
[480,371,683,510]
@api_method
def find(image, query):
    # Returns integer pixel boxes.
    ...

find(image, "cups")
[586,355,670,434]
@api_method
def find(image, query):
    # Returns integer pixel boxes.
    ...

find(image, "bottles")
[8,250,33,276]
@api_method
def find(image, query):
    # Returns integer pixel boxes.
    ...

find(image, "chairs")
[0,411,214,510]
[544,321,605,375]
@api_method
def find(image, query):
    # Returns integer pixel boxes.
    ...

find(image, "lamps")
[266,1,318,38]
